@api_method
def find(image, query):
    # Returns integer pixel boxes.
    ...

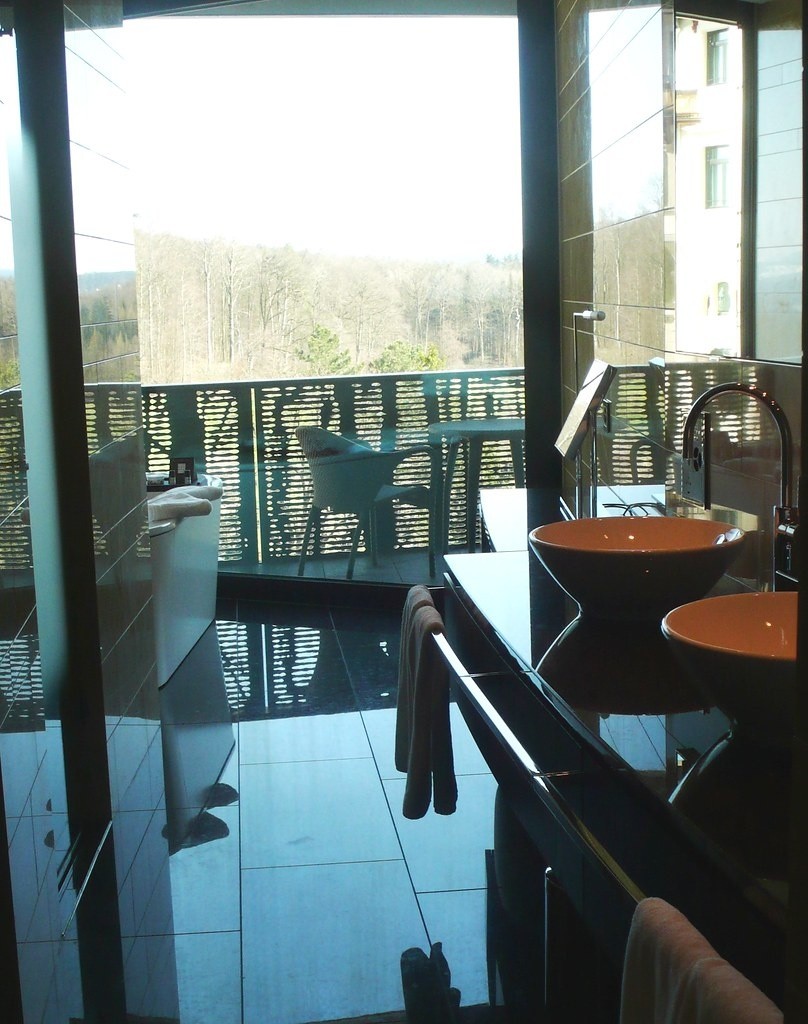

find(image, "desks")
[427,418,527,556]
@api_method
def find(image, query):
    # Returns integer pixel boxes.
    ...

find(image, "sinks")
[660,590,805,721]
[527,517,748,614]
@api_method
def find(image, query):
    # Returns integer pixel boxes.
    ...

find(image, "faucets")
[683,381,799,546]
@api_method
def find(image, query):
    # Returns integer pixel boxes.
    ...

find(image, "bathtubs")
[150,455,223,690]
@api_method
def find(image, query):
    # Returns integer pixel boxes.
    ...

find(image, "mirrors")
[585,0,804,368]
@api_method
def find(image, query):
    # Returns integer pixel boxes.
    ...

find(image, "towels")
[392,581,459,822]
[615,895,788,1024]
[147,484,223,520]
[396,941,462,1012]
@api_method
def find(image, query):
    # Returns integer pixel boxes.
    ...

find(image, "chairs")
[296,426,437,582]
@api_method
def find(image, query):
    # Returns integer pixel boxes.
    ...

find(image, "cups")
[146,474,164,486]
